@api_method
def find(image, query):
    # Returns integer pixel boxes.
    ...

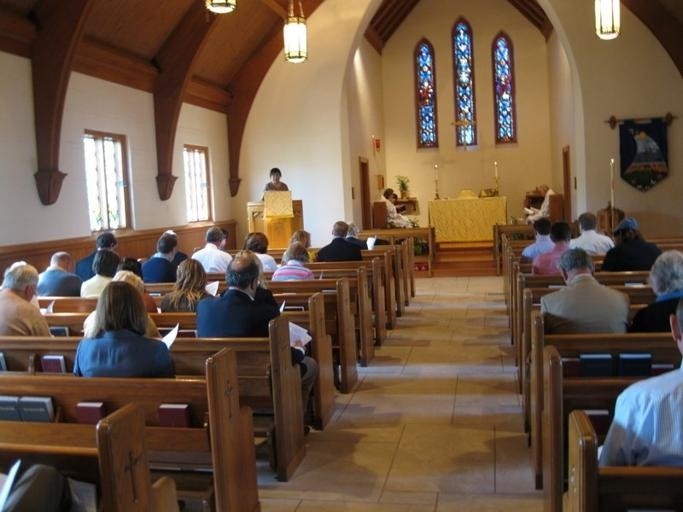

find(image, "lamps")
[281,1,309,64]
[593,0,620,41]
[206,1,236,13]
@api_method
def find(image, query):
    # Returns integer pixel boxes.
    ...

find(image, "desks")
[427,195,506,248]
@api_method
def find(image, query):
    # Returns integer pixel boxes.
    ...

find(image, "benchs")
[0,199,419,512]
[499,191,683,511]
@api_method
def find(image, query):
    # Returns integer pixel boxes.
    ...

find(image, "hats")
[612,216,636,234]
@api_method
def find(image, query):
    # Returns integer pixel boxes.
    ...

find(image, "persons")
[522,184,553,222]
[0,465,73,512]
[34,229,188,297]
[0,261,54,371]
[519,209,682,466]
[380,186,413,229]
[195,255,317,436]
[191,221,370,281]
[261,168,289,202]
[73,280,176,378]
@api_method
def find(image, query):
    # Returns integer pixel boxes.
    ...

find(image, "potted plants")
[394,176,409,199]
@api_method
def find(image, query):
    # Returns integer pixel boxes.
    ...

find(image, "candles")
[435,165,438,179]
[610,159,615,192]
[494,162,497,178]
